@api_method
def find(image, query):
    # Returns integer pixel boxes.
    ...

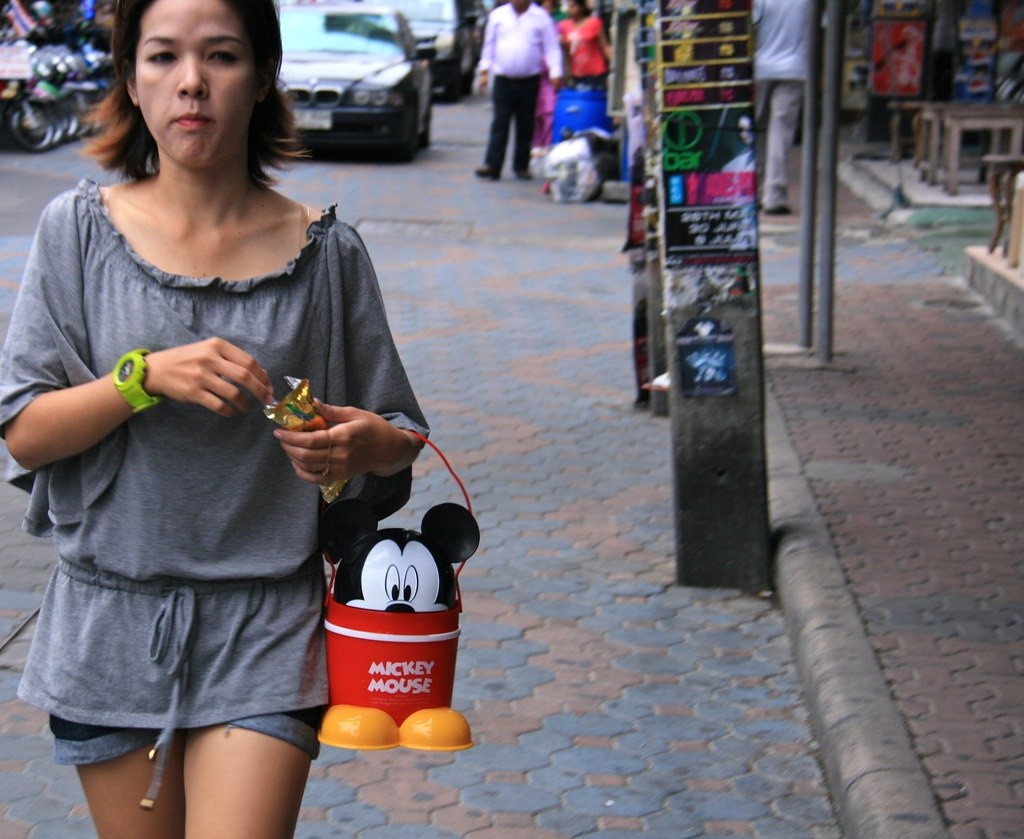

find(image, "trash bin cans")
[550,78,613,146]
[622,104,648,183]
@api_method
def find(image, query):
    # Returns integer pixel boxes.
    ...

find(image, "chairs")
[890,51,1024,271]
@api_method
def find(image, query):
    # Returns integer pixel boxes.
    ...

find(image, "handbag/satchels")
[542,137,601,203]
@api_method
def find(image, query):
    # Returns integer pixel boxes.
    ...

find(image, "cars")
[368,0,480,104]
[277,0,439,162]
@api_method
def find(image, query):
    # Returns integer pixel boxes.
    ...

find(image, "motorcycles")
[0,36,113,156]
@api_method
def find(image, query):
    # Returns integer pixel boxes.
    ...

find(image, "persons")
[472,0,613,181]
[750,0,828,216]
[0,0,431,839]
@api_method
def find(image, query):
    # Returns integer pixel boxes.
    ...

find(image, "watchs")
[111,347,163,414]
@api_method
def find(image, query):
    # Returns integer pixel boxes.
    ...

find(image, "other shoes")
[476,167,501,179]
[764,205,791,214]
[517,167,529,179]
